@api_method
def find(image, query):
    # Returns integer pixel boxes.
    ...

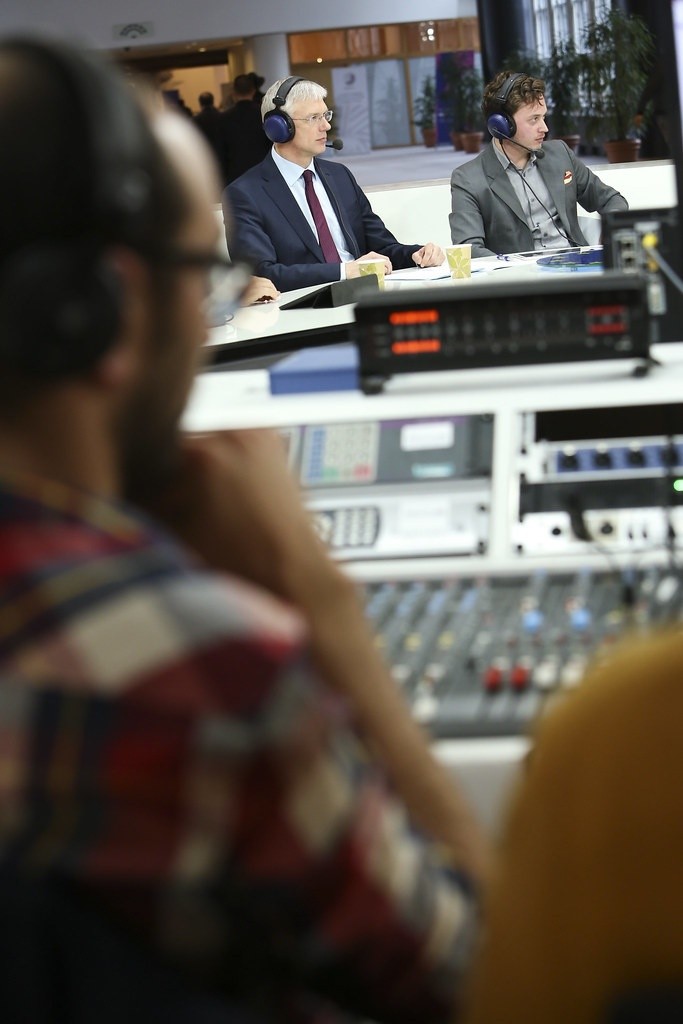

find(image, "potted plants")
[502,4,655,164]
[406,67,486,154]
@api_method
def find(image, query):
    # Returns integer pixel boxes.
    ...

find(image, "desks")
[190,241,612,358]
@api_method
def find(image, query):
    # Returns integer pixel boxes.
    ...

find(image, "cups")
[356,259,387,291]
[444,244,472,279]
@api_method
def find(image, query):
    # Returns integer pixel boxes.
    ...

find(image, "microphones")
[325,139,343,150]
[492,129,545,159]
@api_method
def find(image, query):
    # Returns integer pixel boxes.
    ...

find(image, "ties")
[303,170,342,263]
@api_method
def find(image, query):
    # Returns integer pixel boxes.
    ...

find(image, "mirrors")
[288,50,491,193]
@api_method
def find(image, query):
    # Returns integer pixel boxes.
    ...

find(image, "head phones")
[264,75,310,143]
[487,73,528,139]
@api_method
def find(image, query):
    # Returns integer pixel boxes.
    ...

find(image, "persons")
[221,75,445,294]
[636,66,670,159]
[177,72,273,185]
[0,32,490,1023]
[448,70,630,260]
[459,621,683,1024]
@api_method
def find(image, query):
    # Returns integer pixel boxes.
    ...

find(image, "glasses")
[142,250,253,325]
[291,110,333,127]
